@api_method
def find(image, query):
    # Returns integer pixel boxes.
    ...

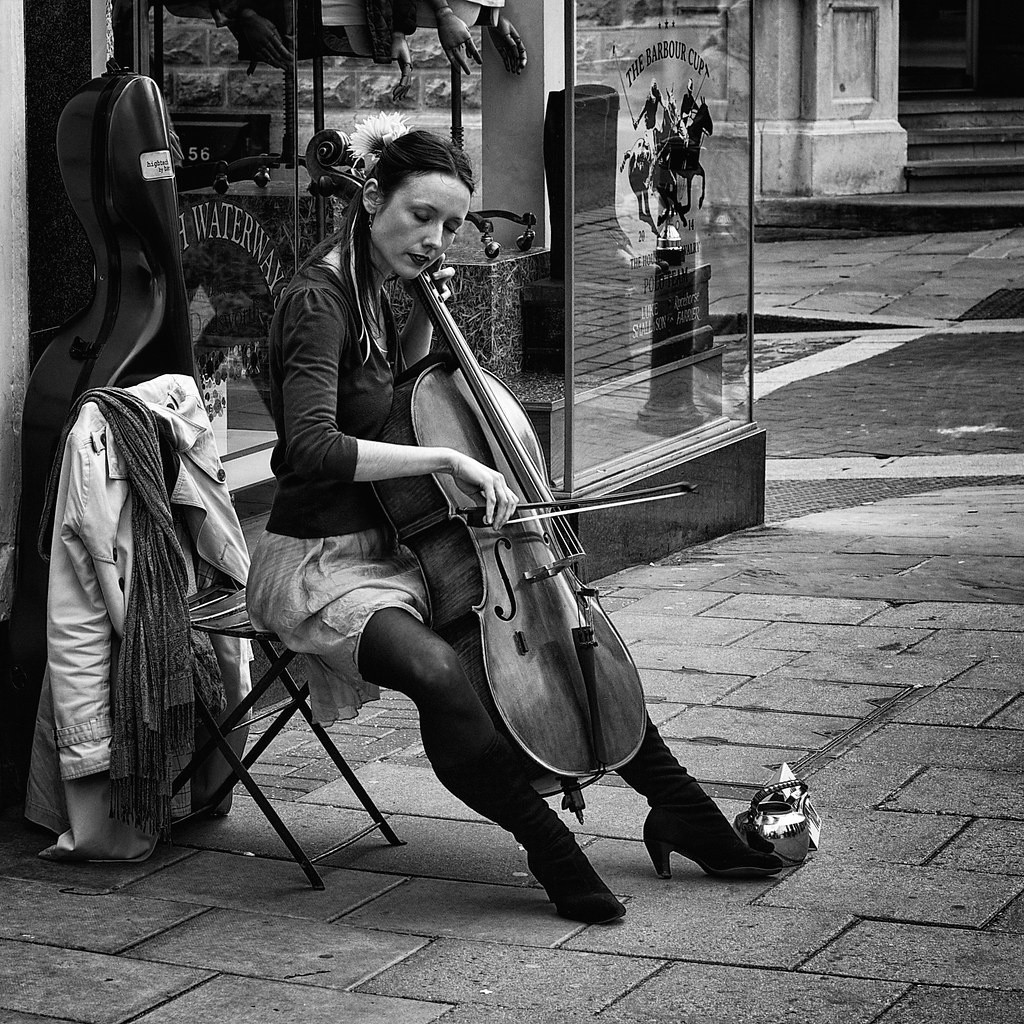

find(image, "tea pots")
[734,779,810,867]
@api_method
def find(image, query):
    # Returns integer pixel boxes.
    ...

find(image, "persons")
[632,77,698,141]
[243,130,784,922]
[415,1,528,76]
[197,0,418,103]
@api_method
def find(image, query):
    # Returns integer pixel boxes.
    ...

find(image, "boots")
[433,734,628,930]
[616,712,782,878]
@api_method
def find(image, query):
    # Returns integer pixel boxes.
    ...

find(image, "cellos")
[303,127,649,823]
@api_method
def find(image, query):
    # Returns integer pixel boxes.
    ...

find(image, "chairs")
[80,371,403,894]
